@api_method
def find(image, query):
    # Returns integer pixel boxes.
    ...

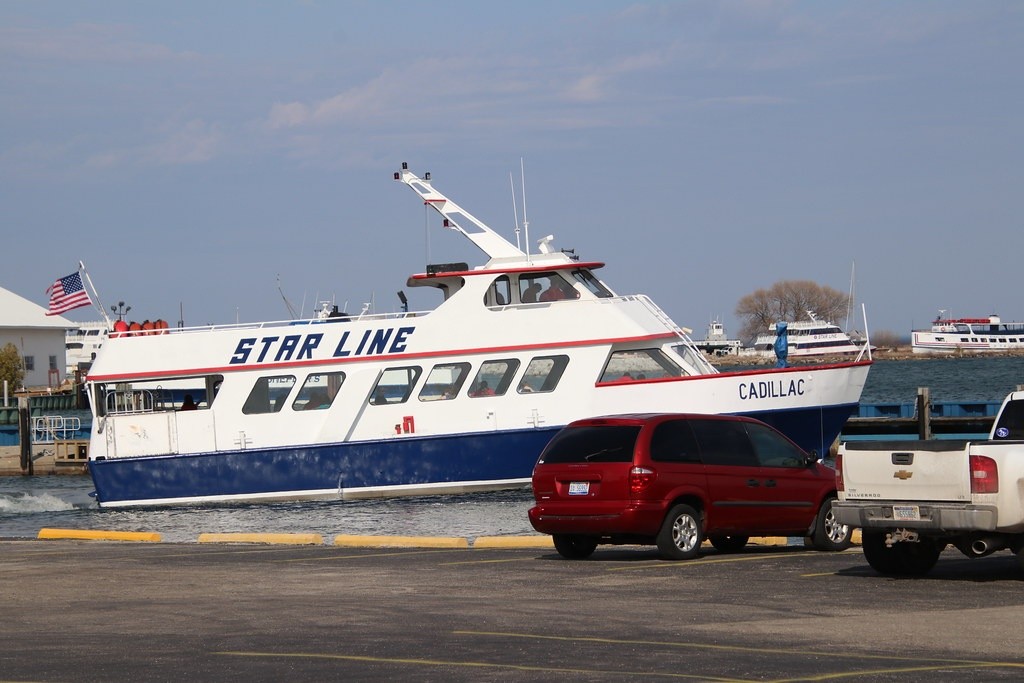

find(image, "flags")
[45,272,92,316]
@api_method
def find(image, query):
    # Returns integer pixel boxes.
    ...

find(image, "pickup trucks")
[831,391,1024,574]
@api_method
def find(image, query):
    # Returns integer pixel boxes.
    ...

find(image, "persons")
[375,387,387,404]
[304,393,330,410]
[471,381,494,396]
[522,283,542,303]
[181,395,201,411]
[564,274,585,299]
[519,381,533,393]
[274,393,287,411]
[539,278,566,300]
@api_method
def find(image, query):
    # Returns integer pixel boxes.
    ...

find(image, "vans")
[528,412,854,560]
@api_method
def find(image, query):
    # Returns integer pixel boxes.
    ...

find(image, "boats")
[689,259,877,356]
[47,159,874,511]
[910,309,1024,353]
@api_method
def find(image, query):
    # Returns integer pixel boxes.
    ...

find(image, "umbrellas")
[775,322,789,368]
